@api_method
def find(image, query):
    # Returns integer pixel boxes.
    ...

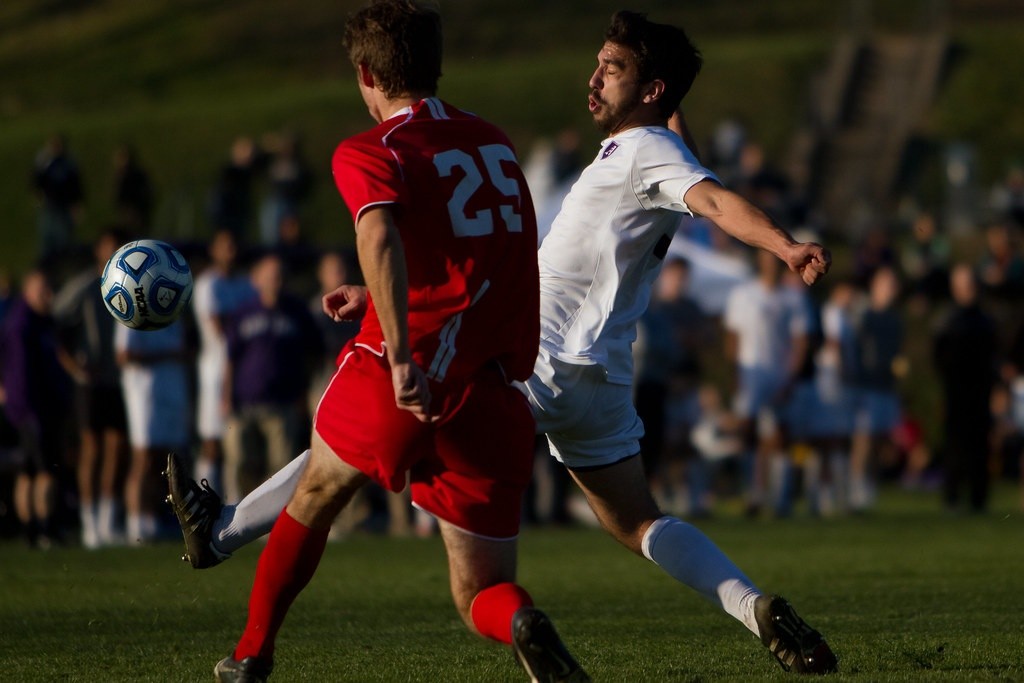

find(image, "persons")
[162,9,843,675]
[213,1,594,683]
[0,129,1024,552]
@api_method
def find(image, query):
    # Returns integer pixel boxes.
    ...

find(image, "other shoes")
[82,527,98,549]
[98,525,117,544]
[130,523,147,546]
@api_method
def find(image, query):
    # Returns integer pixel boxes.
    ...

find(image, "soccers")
[101,238,195,332]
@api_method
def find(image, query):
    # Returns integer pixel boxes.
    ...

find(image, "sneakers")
[754,592,838,676]
[161,451,233,569]
[511,607,591,683]
[213,656,272,683]
